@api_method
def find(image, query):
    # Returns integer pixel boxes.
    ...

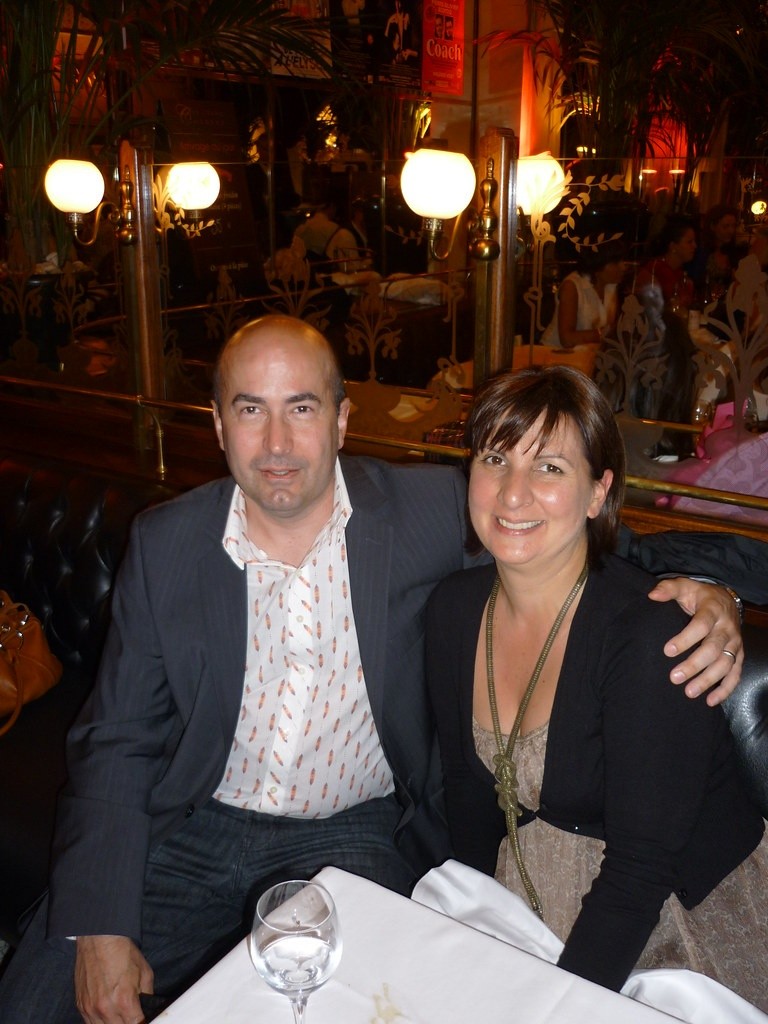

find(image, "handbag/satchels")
[0,590,62,736]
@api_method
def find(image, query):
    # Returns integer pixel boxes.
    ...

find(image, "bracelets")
[716,584,744,625]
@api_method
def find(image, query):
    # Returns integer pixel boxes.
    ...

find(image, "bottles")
[701,276,711,306]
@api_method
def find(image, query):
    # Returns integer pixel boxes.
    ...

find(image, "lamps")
[399,147,500,262]
[153,162,220,252]
[514,152,565,261]
[44,159,138,249]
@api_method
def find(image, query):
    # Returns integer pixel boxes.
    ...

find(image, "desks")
[430,343,593,390]
[345,379,701,479]
[149,867,686,1024]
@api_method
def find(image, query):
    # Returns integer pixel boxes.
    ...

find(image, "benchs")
[0,448,768,947]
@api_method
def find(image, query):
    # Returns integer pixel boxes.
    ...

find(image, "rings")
[720,650,736,664]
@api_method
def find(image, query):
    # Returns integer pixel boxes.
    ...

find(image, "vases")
[23,275,73,372]
[0,275,23,362]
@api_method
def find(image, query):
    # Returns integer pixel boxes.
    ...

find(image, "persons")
[421,365,768,1021]
[0,312,747,1024]
[0,190,768,448]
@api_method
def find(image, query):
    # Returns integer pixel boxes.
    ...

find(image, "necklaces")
[486,554,589,922]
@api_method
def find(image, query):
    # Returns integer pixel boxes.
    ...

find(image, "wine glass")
[250,880,343,1024]
